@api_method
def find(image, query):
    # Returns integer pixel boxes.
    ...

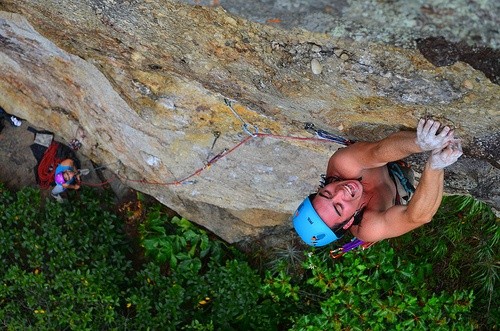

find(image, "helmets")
[54,172,66,185]
[290,192,345,247]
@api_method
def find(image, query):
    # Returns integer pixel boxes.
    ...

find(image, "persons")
[55,159,81,190]
[291,118,463,249]
